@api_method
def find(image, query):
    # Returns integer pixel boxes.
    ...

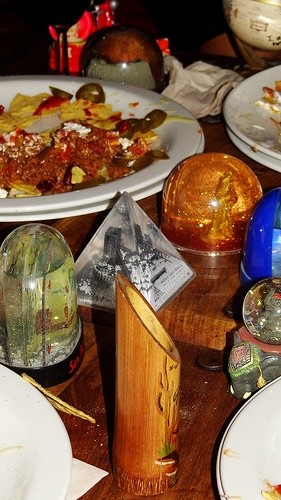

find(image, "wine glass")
[41,0,86,76]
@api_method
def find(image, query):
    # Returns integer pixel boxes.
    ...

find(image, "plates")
[222,63,281,174]
[0,362,73,500]
[0,75,206,223]
[216,377,281,500]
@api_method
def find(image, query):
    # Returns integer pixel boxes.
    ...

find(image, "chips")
[0,91,159,198]
